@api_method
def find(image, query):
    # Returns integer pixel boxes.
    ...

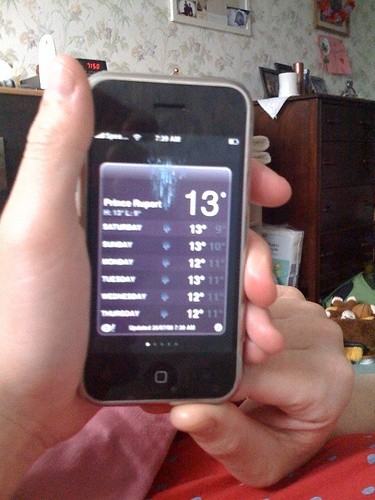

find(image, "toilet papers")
[275,72,300,97]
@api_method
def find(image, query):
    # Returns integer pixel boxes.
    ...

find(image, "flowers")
[320,0,356,23]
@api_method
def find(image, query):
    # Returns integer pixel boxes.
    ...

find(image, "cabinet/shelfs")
[253,94,375,302]
[0,76,43,215]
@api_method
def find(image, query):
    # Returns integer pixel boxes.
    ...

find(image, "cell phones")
[77,70,253,406]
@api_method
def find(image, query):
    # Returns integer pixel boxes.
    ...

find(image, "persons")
[0,55,375,500]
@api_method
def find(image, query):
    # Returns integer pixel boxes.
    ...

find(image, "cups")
[279,73,298,97]
[293,62,310,96]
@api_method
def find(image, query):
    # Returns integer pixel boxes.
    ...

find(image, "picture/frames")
[259,67,279,98]
[170,0,252,37]
[313,0,350,37]
[310,75,327,95]
[274,63,292,74]
